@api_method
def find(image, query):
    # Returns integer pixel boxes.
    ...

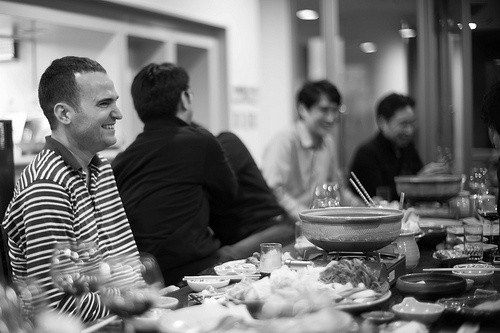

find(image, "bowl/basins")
[187,279,230,292]
[396,273,467,301]
[452,263,495,287]
[391,302,445,328]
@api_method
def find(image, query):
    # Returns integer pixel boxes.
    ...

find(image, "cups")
[464,224,483,260]
[310,183,341,209]
[259,243,282,277]
[468,168,489,195]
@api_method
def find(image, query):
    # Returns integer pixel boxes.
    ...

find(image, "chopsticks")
[182,275,261,280]
[423,268,500,271]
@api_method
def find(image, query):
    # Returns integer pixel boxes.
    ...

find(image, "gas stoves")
[399,198,461,219]
[285,247,407,290]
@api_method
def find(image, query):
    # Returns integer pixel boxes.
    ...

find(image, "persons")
[2,56,180,327]
[260,78,383,223]
[445,83,500,228]
[190,120,301,262]
[348,91,449,211]
[109,62,239,287]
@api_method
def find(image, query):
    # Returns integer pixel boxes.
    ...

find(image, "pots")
[299,207,404,253]
[394,176,462,201]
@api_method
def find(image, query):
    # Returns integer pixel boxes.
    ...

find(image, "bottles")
[379,229,422,269]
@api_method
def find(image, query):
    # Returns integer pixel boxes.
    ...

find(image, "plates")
[416,217,500,263]
[125,259,500,333]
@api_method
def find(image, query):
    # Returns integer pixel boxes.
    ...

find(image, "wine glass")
[475,187,500,257]
